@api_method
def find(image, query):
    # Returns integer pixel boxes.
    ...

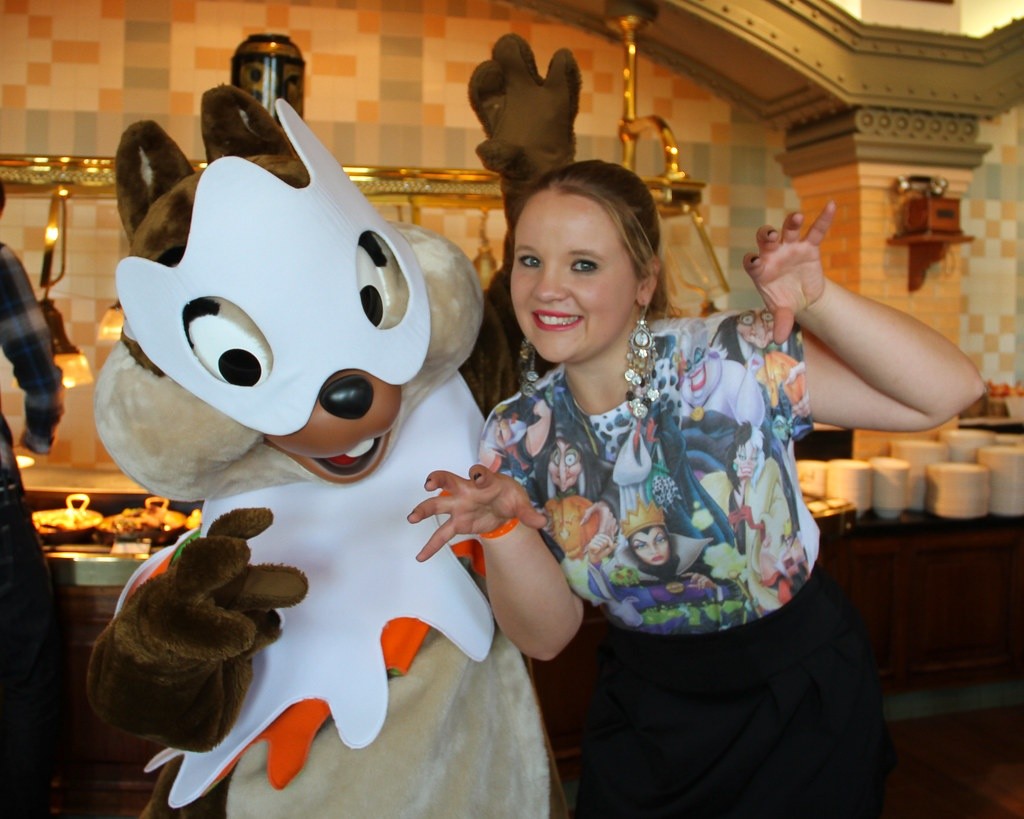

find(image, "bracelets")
[438,490,519,538]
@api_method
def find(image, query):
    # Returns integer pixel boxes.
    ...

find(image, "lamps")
[472,207,496,291]
[97,300,125,344]
[15,195,94,389]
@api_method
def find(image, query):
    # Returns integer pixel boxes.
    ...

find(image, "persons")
[0,176,89,789]
[409,160,987,818]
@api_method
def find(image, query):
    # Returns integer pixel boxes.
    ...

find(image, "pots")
[98,495,186,546]
[31,494,104,544]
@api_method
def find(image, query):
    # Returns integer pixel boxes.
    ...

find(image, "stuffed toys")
[84,32,583,819]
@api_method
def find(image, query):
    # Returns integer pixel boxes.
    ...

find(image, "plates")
[824,428,1024,520]
